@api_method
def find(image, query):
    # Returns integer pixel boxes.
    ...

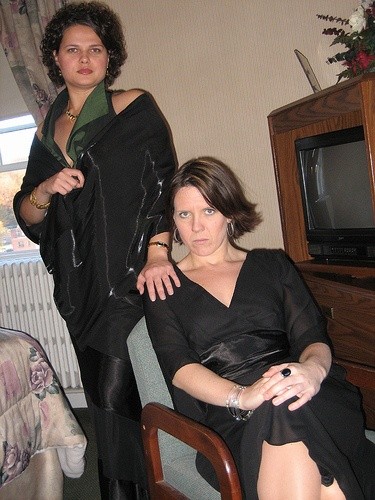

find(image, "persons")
[13,2,179,500]
[145,157,375,500]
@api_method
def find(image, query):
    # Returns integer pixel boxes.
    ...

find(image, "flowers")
[316,0,375,85]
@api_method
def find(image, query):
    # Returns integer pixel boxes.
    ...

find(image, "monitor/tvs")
[296,125,375,267]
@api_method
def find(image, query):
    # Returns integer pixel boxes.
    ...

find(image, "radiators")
[0,262,87,408]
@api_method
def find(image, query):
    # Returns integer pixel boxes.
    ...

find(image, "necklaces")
[66,110,80,120]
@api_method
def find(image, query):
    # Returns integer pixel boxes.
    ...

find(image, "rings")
[281,368,292,375]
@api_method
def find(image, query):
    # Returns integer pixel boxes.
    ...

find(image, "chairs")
[128,315,241,500]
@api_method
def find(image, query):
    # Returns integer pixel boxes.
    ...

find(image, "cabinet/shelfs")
[267,71,374,434]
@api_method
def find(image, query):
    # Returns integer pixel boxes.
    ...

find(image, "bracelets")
[29,187,51,209]
[147,241,170,250]
[226,385,253,421]
